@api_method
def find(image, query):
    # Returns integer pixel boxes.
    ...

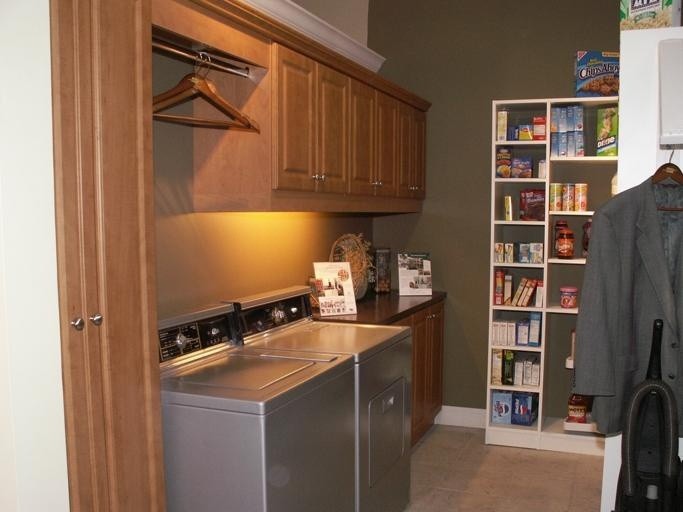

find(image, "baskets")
[327,233,368,300]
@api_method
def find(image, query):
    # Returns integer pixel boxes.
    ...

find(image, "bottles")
[554,219,569,256]
[581,218,592,258]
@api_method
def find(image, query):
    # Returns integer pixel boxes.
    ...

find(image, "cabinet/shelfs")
[484,94,618,457]
[391,300,444,447]
[349,59,398,211]
[50,0,169,511]
[396,86,433,211]
[195,40,349,212]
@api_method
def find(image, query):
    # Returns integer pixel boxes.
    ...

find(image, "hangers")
[152,48,259,131]
[594,145,682,214]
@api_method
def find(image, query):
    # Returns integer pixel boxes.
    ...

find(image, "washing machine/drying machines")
[233,284,412,512]
[157,301,356,512]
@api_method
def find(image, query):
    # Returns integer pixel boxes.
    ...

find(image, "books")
[516,280,530,307]
[502,275,512,306]
[512,278,527,306]
[494,269,504,305]
[535,280,543,307]
[521,279,537,306]
